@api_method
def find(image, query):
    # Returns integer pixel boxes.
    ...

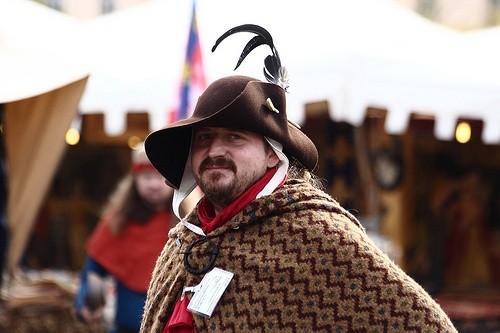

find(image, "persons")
[140,75,462,333]
[73,141,179,333]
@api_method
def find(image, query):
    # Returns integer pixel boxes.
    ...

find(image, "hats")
[146,23,319,189]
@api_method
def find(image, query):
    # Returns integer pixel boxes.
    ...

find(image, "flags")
[174,6,207,123]
